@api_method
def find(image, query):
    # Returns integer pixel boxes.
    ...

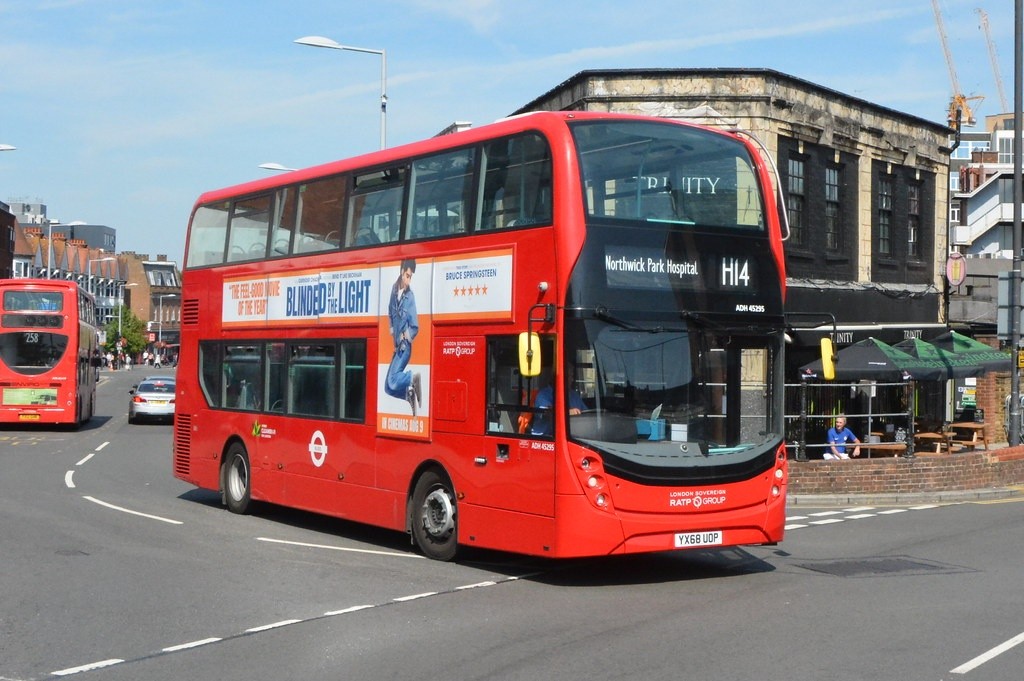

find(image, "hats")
[125,354,129,357]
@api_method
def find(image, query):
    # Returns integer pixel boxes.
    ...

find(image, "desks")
[950,423,988,451]
[914,433,957,455]
[846,443,906,457]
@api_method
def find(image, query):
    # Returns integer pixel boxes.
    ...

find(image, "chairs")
[271,399,282,411]
[144,386,154,392]
[517,386,538,436]
[232,227,381,262]
[507,216,536,228]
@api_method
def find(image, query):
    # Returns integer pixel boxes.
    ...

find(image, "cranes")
[973,7,1008,113]
[931,0,986,133]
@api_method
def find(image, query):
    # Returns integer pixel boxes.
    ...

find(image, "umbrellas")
[927,329,1012,440]
[892,336,985,453]
[799,336,942,459]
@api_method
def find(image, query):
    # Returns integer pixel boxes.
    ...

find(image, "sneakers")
[411,374,420,404]
[407,389,417,417]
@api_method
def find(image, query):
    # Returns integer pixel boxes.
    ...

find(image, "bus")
[171,112,842,562]
[0,278,97,430]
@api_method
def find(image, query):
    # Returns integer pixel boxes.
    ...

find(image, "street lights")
[46,220,87,280]
[291,35,387,151]
[118,282,140,336]
[159,294,176,342]
[88,257,116,294]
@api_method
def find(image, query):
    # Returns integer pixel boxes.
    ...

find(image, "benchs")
[914,440,979,455]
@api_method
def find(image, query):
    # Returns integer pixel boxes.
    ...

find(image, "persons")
[822,413,861,460]
[96,347,178,370]
[531,360,589,435]
[384,258,422,416]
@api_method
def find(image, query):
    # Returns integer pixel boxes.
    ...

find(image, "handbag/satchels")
[125,364,132,370]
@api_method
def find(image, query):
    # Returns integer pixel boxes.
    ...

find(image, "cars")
[127,376,176,425]
